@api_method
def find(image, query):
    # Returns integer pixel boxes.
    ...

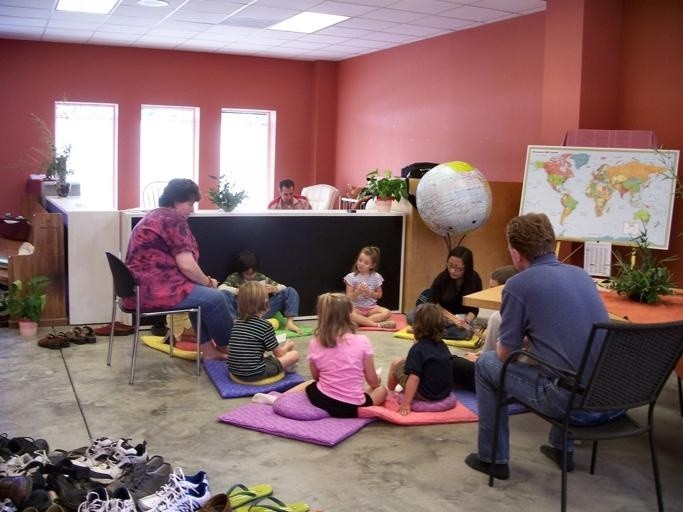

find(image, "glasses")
[445,263,466,272]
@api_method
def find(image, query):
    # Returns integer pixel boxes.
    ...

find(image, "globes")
[415,161,494,252]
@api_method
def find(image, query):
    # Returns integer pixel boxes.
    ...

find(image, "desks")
[462,276,683,419]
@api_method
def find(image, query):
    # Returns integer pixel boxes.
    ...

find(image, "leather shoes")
[96,322,135,336]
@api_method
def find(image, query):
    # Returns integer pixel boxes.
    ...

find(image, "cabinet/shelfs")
[41,182,120,331]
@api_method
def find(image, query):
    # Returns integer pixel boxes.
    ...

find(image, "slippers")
[222,481,309,512]
[38,326,96,349]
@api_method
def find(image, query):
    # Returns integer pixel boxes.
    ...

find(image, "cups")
[361,280,369,287]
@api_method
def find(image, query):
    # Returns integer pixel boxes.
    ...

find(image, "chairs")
[488,320,683,512]
[142,181,168,209]
[106,252,201,385]
[301,184,340,209]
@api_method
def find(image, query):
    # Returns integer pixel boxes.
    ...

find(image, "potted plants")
[206,174,248,212]
[11,90,77,196]
[608,228,679,304]
[360,168,408,212]
[2,276,51,338]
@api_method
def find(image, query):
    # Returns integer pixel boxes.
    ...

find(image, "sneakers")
[0,435,230,512]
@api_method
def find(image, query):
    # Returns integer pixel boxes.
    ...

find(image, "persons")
[226,280,300,382]
[342,245,397,328]
[120,177,234,362]
[463,211,627,480]
[405,245,483,340]
[387,302,455,417]
[267,179,312,210]
[463,264,529,364]
[304,292,388,419]
[216,248,304,336]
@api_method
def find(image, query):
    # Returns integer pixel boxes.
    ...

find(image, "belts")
[546,375,585,395]
